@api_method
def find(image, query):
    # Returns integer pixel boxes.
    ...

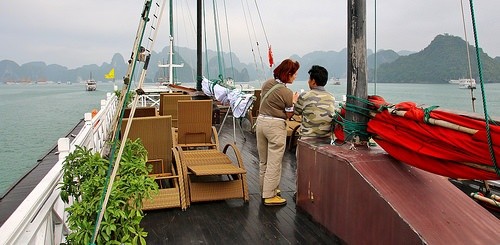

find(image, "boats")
[448,78,475,89]
[84,71,96,91]
[329,77,340,86]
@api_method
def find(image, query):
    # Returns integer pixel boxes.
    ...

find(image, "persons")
[292,65,336,203]
[255,59,300,205]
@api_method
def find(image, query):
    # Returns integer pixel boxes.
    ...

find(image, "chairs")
[121,90,260,211]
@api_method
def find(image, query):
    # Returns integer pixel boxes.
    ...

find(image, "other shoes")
[262,189,280,196]
[264,196,287,206]
